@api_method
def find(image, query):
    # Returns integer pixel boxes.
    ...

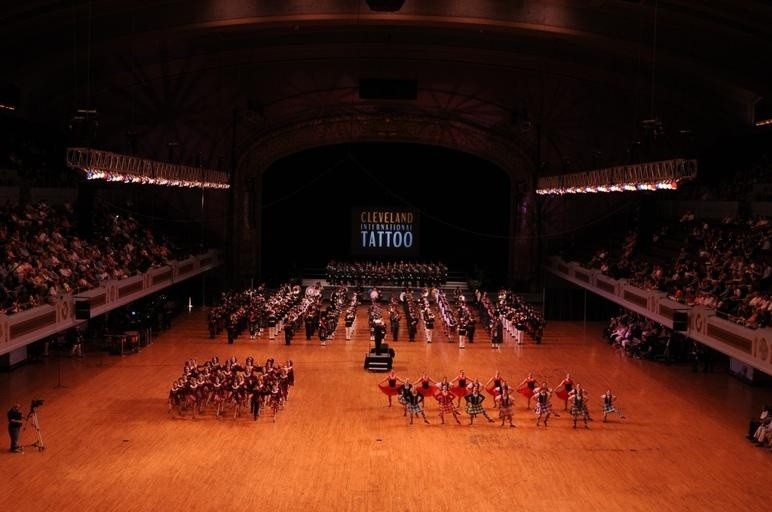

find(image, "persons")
[6,403,25,452]
[207,282,548,347]
[377,369,626,431]
[169,355,294,423]
[325,262,453,287]
[1,180,180,356]
[373,319,385,355]
[565,179,772,441]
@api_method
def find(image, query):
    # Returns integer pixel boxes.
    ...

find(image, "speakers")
[76,301,90,320]
[673,312,688,331]
[18,409,44,450]
